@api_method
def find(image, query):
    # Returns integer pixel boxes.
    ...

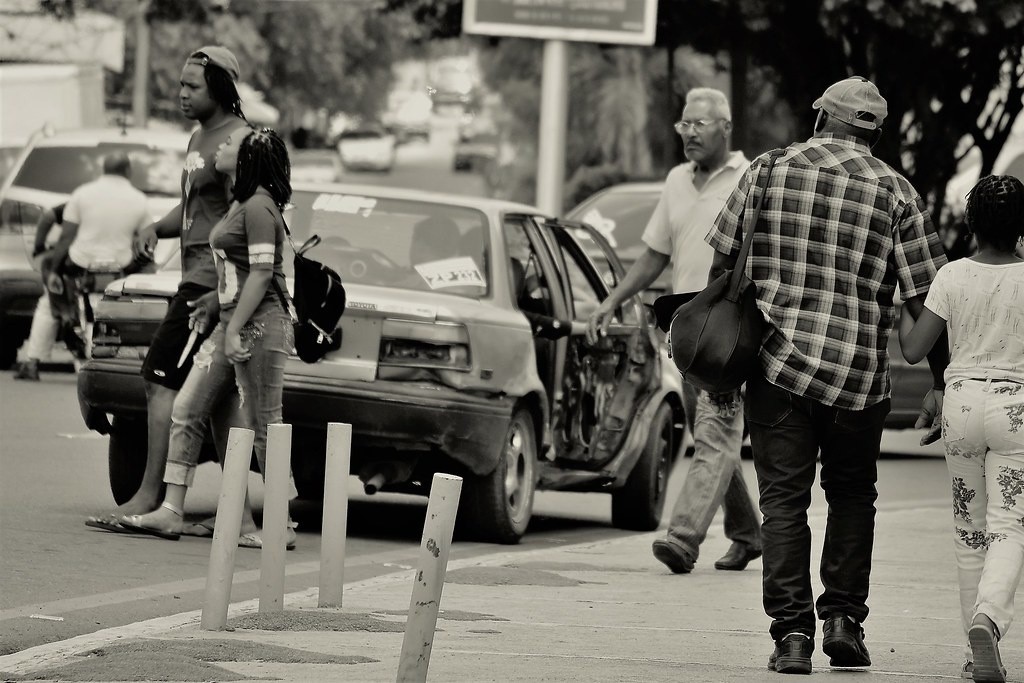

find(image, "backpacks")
[243,193,346,364]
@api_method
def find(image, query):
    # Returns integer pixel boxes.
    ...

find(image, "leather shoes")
[652,540,696,574]
[714,541,763,570]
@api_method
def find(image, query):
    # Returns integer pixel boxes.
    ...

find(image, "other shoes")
[14,358,41,383]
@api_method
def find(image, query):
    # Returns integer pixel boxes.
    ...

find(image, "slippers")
[182,522,214,537]
[85,513,137,534]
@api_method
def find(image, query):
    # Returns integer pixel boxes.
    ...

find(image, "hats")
[187,45,239,84]
[812,76,888,130]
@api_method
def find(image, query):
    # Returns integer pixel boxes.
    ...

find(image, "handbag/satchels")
[665,148,788,395]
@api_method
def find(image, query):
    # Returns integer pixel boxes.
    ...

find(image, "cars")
[564,180,934,424]
[0,100,691,543]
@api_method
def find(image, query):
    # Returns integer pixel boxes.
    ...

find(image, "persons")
[703,76,948,675]
[13,153,156,381]
[585,87,762,574]
[84,46,257,538]
[119,126,298,551]
[898,175,1024,683]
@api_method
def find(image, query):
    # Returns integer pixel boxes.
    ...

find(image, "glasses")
[675,117,728,134]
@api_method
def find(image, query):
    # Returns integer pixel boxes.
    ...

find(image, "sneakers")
[822,612,872,666]
[768,626,816,673]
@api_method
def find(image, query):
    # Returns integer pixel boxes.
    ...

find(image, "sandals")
[238,519,299,551]
[119,501,184,541]
[960,660,975,678]
[967,624,1008,683]
